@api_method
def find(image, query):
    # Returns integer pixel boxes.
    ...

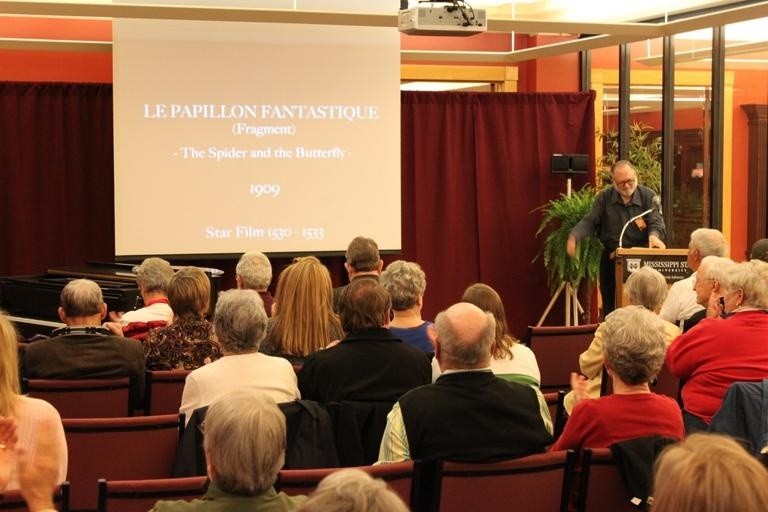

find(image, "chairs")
[604,354,682,403]
[529,385,565,423]
[327,398,392,466]
[94,470,214,510]
[145,360,200,414]
[187,395,324,467]
[580,445,681,511]
[712,378,768,455]
[286,356,303,373]
[522,325,599,385]
[32,414,192,509]
[436,445,574,507]
[21,375,137,414]
[274,454,421,509]
[2,476,75,512]
